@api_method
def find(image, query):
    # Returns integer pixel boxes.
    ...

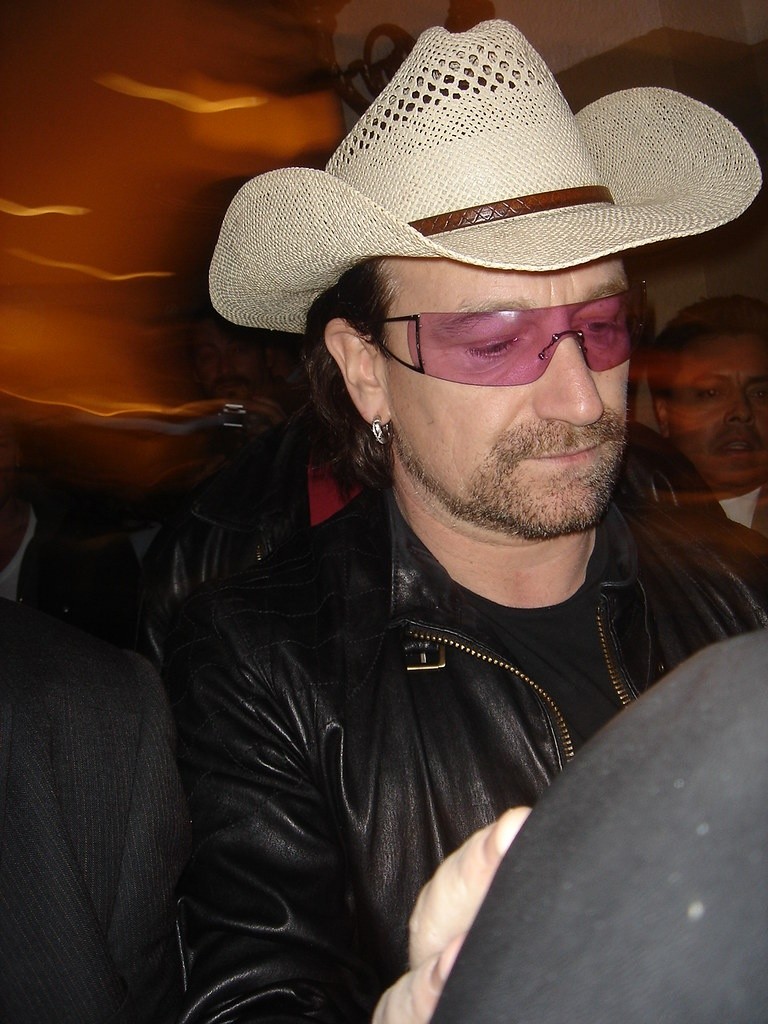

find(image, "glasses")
[359,280,649,387]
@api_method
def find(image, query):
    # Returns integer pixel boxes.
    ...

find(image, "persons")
[0,174,768,1024]
[168,18,768,1024]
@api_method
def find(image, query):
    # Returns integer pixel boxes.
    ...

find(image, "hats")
[208,20,763,333]
